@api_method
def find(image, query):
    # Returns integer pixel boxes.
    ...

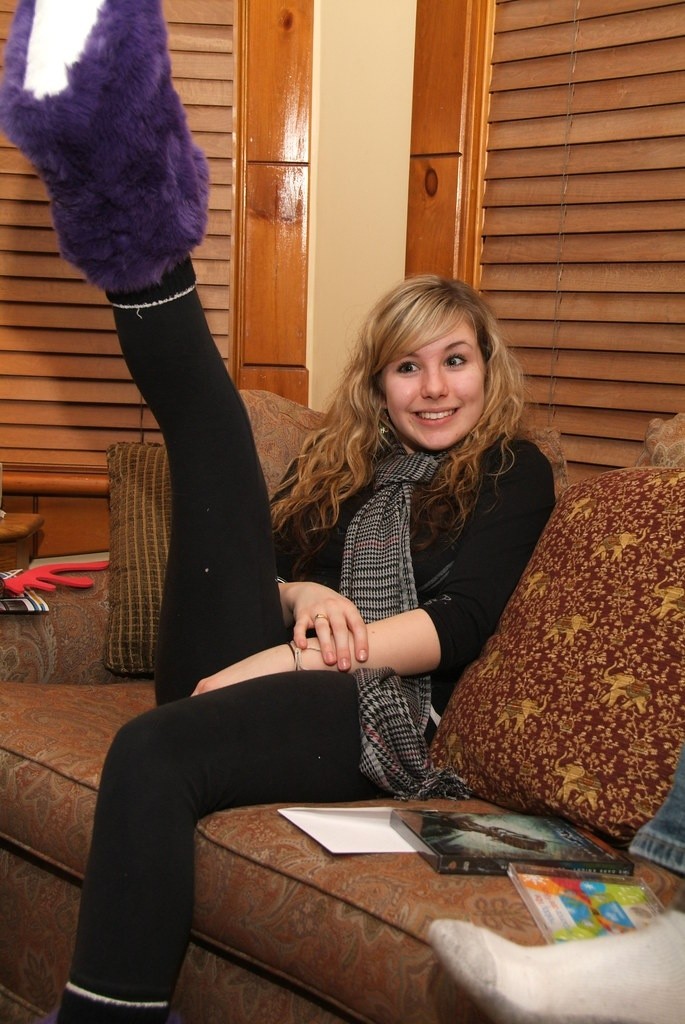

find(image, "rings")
[314,614,327,619]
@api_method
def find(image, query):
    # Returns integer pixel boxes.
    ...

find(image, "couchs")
[0,570,683,1024]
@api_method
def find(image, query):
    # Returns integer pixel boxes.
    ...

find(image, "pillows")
[431,467,685,849]
[106,439,174,673]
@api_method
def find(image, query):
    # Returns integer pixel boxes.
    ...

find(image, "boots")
[0,0,210,294]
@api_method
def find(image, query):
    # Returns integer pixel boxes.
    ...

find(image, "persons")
[1,1,555,1024]
[425,742,685,1024]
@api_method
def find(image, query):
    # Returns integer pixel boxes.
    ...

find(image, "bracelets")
[286,640,308,671]
